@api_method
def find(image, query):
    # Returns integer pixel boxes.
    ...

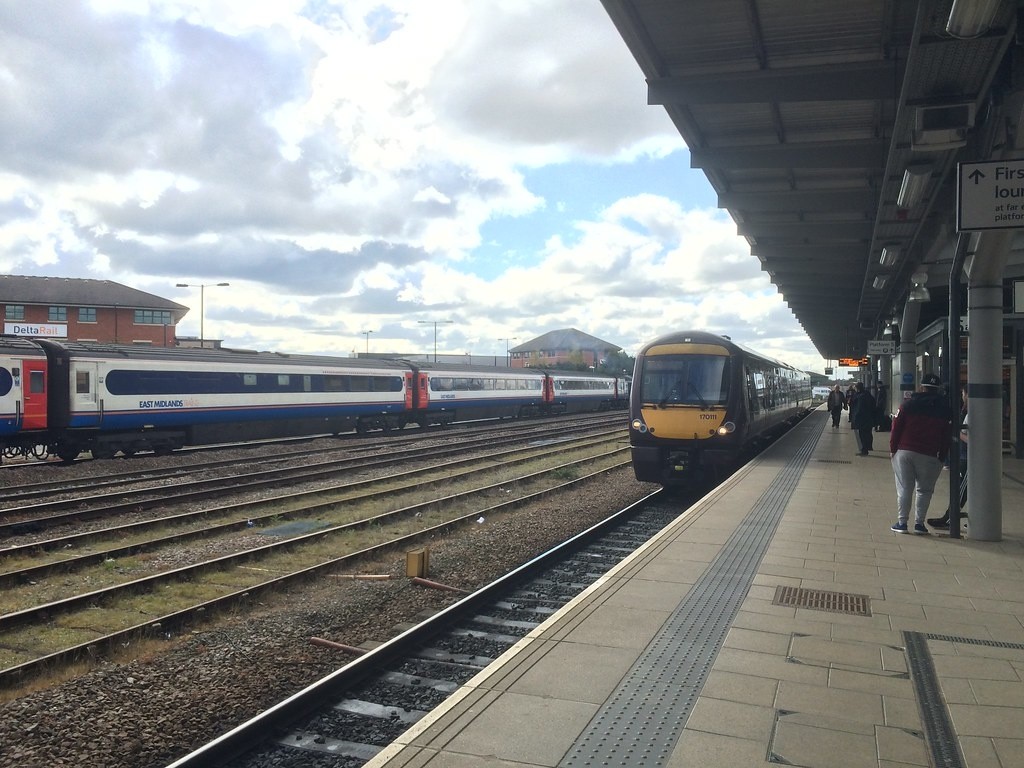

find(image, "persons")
[826,377,887,457]
[927,381,972,529]
[888,375,949,534]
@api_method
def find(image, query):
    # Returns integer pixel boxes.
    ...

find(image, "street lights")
[418,320,454,363]
[176,283,230,349]
[498,338,517,368]
[363,330,372,356]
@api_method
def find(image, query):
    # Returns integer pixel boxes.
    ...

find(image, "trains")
[627,330,813,494]
[0,339,634,463]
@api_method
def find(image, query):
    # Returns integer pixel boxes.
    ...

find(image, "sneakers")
[914,523,928,533]
[890,522,908,533]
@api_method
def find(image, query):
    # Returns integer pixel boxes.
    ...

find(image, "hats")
[921,374,940,387]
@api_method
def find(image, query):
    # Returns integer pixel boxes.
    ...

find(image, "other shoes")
[832,424,839,428]
[855,450,869,456]
[927,519,949,529]
